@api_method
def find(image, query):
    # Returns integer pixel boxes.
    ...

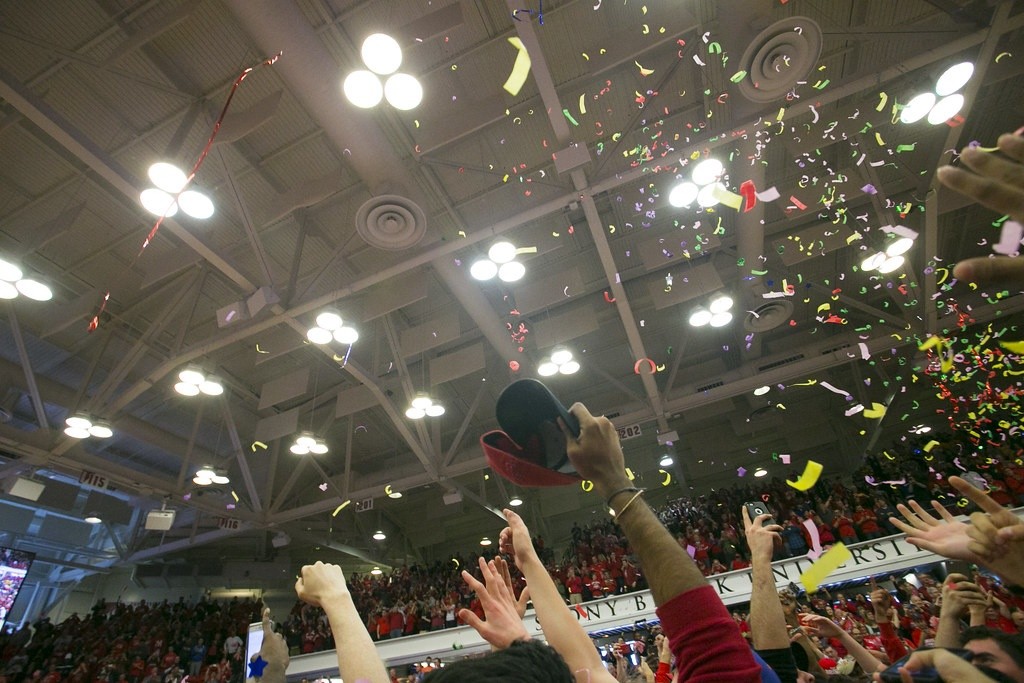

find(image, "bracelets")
[607,486,638,506]
[661,652,670,656]
[613,490,645,522]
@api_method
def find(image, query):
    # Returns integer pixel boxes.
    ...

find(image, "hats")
[480,379,585,487]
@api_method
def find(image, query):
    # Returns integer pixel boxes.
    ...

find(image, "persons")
[1,133,1024,683]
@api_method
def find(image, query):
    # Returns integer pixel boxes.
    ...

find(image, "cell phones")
[945,558,977,593]
[780,581,799,605]
[619,643,635,654]
[745,501,776,527]
[881,645,975,683]
[244,620,275,678]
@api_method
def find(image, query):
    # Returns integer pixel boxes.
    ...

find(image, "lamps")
[479,536,492,546]
[668,154,727,210]
[371,566,382,575]
[468,235,526,283]
[193,463,229,486]
[373,530,386,540]
[658,454,674,467]
[405,392,445,419]
[290,430,329,456]
[510,495,523,506]
[859,235,914,274]
[306,305,359,345]
[173,364,225,398]
[687,291,734,328]
[537,344,580,376]
[85,511,102,524]
[63,410,114,442]
[899,58,975,126]
